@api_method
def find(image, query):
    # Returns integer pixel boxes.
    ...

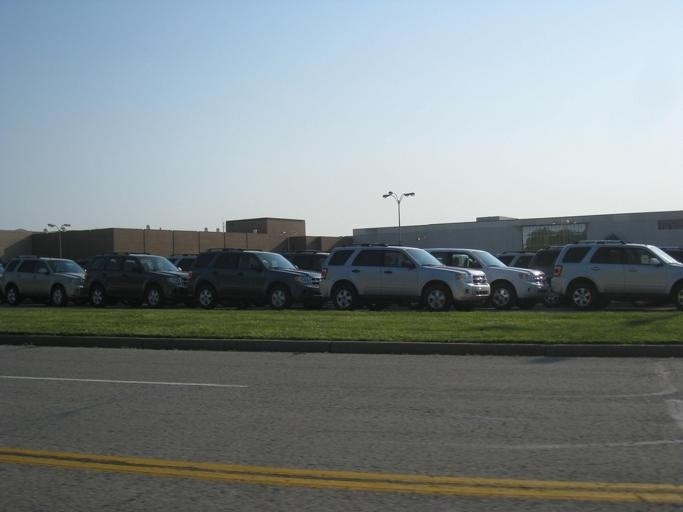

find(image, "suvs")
[550,240,683,311]
[0,255,86,308]
[425,247,549,311]
[191,247,321,310]
[319,243,492,312]
[82,251,193,309]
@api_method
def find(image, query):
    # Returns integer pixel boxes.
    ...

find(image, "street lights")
[279,231,298,251]
[43,223,71,258]
[382,191,415,246]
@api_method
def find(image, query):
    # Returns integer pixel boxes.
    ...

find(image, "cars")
[0,240,683,311]
